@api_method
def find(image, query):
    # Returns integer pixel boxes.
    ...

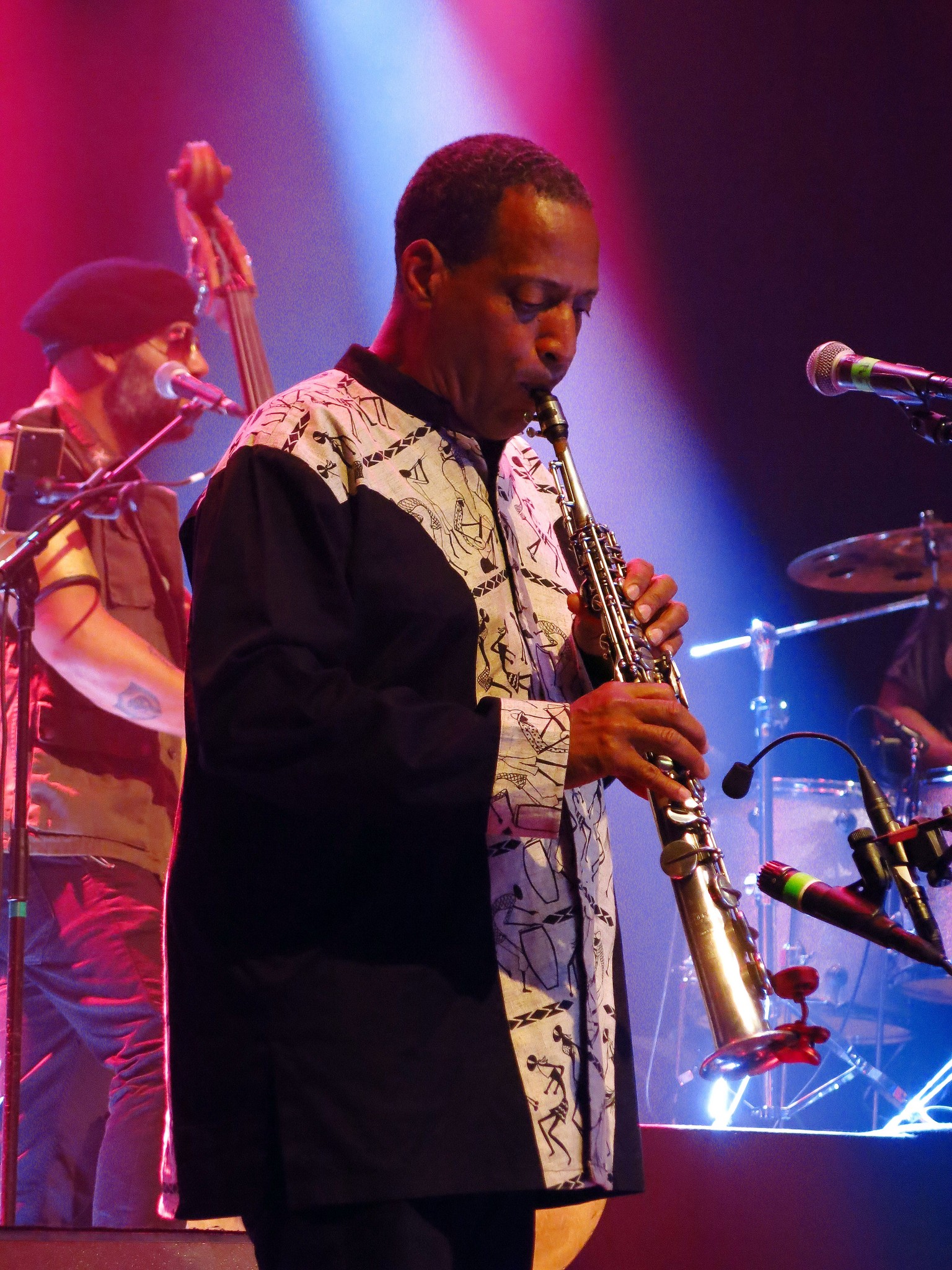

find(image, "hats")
[23,259,198,346]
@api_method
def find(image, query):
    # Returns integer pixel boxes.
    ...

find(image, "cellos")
[165,136,269,412]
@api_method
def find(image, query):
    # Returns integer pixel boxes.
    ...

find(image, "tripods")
[688,528,945,1132]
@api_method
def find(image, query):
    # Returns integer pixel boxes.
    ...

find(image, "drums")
[890,760,952,1003]
[695,774,909,1047]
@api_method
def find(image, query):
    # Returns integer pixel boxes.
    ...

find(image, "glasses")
[143,326,192,355]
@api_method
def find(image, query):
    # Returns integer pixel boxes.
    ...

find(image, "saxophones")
[523,380,833,1072]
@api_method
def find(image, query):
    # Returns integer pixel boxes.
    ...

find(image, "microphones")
[875,708,930,754]
[154,359,248,420]
[756,860,947,967]
[722,732,895,838]
[805,340,952,401]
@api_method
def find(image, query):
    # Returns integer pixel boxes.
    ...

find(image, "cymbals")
[785,515,952,592]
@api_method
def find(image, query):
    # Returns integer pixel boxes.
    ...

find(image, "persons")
[875,587,952,771]
[160,132,710,1269]
[2,258,210,1227]
